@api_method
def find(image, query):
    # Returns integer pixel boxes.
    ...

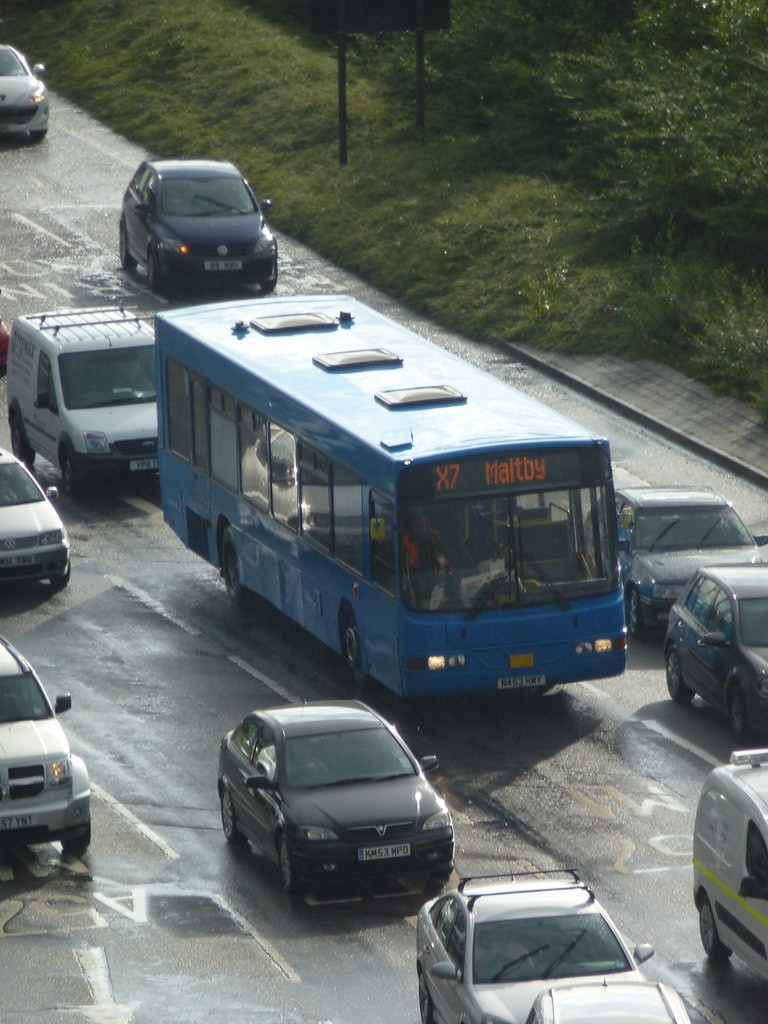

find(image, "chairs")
[458,498,551,552]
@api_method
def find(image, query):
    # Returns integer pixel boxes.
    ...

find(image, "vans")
[693,747,768,977]
[7,305,158,489]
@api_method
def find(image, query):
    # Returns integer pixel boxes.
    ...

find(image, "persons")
[407,517,452,599]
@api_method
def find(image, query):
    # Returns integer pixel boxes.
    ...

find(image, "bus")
[153,293,630,697]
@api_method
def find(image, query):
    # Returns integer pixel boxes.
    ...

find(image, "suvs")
[0,634,91,850]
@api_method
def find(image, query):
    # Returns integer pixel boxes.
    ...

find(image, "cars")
[585,484,768,632]
[0,447,71,585]
[416,867,654,1024]
[119,160,278,291]
[0,45,49,138]
[664,565,768,731]
[527,978,692,1024]
[217,697,455,892]
